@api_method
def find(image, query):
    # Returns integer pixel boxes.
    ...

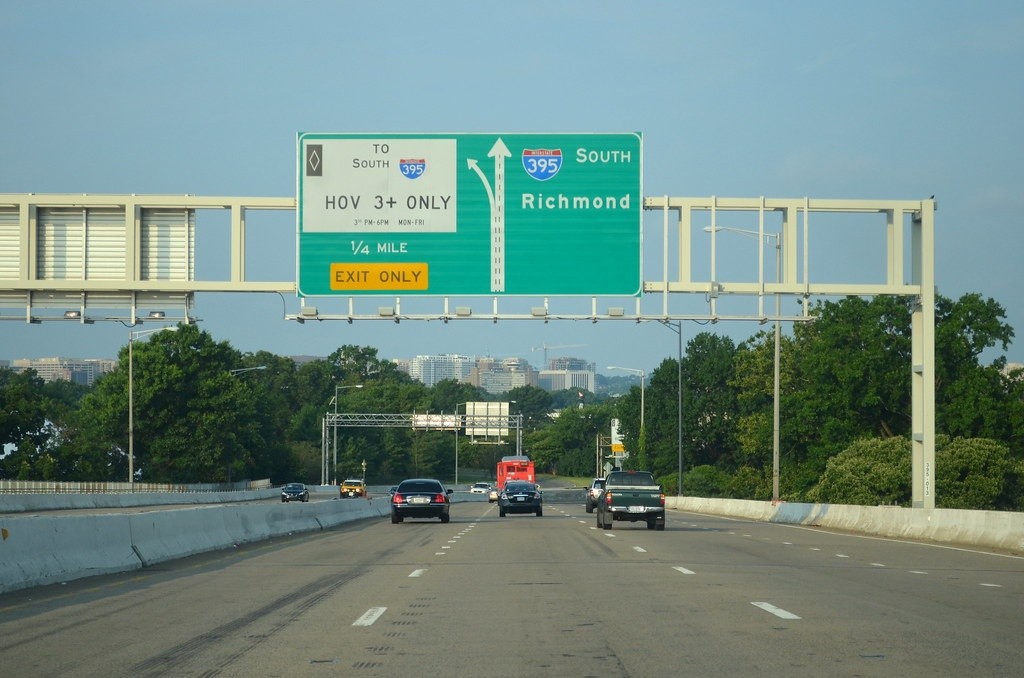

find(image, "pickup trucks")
[596,469,666,530]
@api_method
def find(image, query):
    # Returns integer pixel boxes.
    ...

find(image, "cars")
[387,486,398,498]
[470,482,492,493]
[390,478,454,524]
[489,486,498,503]
[497,480,544,518]
[281,482,309,502]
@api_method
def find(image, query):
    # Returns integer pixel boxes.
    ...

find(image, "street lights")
[128,325,179,482]
[637,315,684,496]
[607,366,645,429]
[228,365,267,482]
[705,224,782,502]
[333,385,364,484]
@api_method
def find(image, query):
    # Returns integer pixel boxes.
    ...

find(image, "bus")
[496,455,535,506]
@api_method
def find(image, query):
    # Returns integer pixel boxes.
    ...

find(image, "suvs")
[583,477,606,514]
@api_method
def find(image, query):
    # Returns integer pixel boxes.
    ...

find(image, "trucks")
[339,479,367,499]
